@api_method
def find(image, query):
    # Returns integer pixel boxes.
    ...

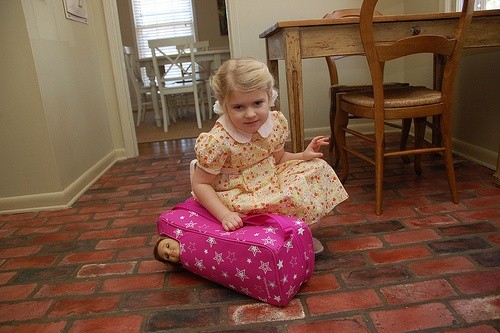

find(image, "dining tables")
[137,48,232,130]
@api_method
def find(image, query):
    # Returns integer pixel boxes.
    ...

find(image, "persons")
[190,56,350,256]
[151,235,181,265]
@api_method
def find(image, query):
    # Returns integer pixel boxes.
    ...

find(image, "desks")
[258,8,500,164]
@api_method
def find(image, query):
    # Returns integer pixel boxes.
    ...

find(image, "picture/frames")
[217,0,229,35]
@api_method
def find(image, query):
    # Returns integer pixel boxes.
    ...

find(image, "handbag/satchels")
[160,197,315,305]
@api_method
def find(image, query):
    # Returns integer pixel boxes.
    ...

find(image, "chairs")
[322,7,427,171]
[175,40,210,117]
[123,45,175,126]
[334,0,475,215]
[147,34,207,133]
